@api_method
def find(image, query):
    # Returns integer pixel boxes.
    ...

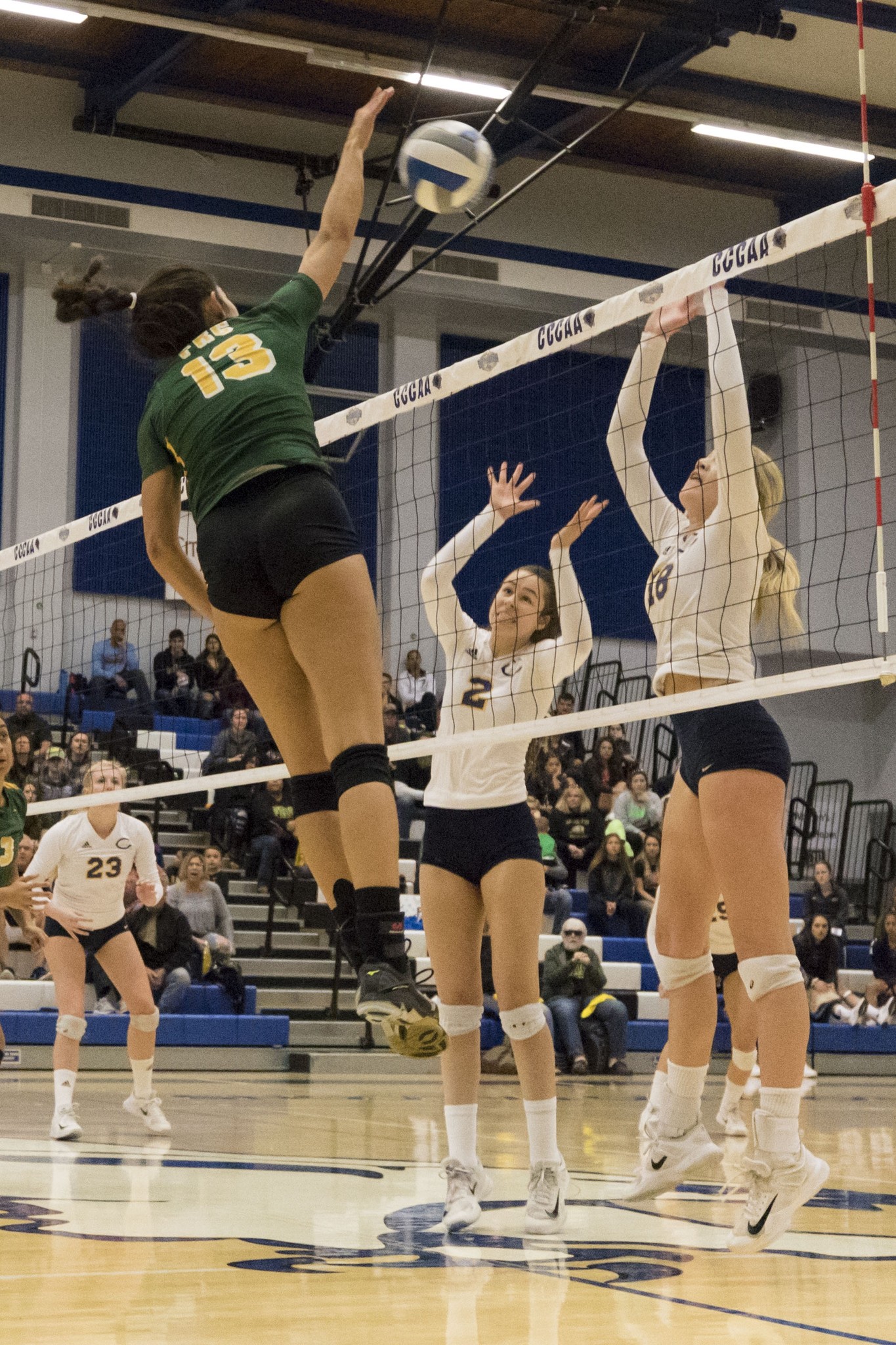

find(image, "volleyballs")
[397,118,497,214]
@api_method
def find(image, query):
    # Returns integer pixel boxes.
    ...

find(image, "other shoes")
[799,1077,817,1099]
[751,1064,760,1076]
[742,1078,760,1100]
[803,1064,817,1077]
[607,1061,634,1076]
[572,1060,588,1075]
[0,966,54,981]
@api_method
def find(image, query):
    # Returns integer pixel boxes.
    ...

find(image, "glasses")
[564,930,582,937]
[611,727,621,731]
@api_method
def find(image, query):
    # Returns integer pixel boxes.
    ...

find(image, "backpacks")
[480,1035,518,1074]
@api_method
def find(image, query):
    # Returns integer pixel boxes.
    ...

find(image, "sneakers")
[123,1090,172,1132]
[716,1108,748,1137]
[50,1103,83,1140]
[354,937,451,1058]
[847,997,868,1028]
[638,1105,660,1142]
[871,995,896,1027]
[442,1150,569,1235]
[93,997,129,1014]
[622,1121,830,1255]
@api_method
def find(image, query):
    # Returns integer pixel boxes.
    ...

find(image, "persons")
[606,275,832,1257]
[397,650,437,737]
[0,716,52,1062]
[419,461,610,1234]
[153,629,199,718]
[866,907,896,1026]
[85,619,156,716]
[639,892,818,1136]
[51,84,451,1063]
[792,912,896,1029]
[24,759,173,1140]
[803,860,850,967]
[540,917,634,1076]
[0,618,679,1018]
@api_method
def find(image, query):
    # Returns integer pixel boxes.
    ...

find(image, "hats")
[45,747,65,761]
[170,629,183,638]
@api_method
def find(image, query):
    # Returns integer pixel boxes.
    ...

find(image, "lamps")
[0,0,88,24]
[306,49,512,100]
[689,120,875,163]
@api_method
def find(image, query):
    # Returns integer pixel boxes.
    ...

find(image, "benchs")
[0,691,896,1075]
